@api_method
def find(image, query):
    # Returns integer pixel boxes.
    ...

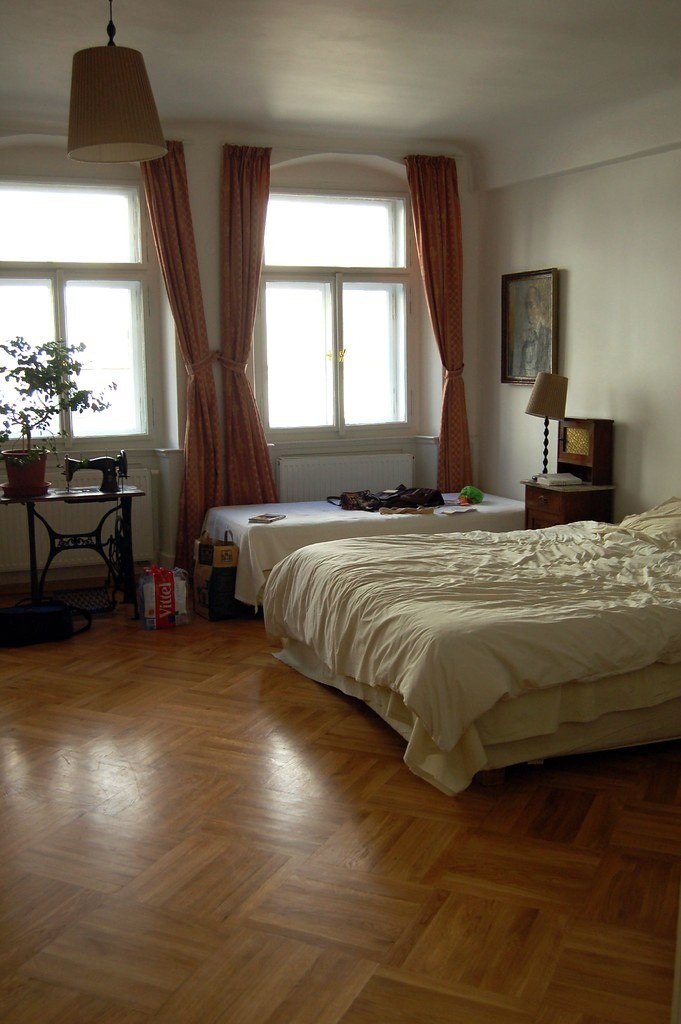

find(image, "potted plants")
[0,335,117,496]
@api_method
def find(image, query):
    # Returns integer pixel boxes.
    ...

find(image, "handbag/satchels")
[0,595,92,649]
[326,489,381,511]
[188,529,239,621]
[137,565,187,630]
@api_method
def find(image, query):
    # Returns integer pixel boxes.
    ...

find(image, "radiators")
[0,466,154,572]
[275,449,415,502]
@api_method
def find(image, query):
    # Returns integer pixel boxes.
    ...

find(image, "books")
[249,514,286,523]
[537,473,582,485]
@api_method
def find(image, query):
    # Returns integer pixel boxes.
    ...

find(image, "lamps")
[525,372,568,479]
[66,0,168,164]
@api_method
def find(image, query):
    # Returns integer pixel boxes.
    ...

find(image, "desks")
[0,485,146,622]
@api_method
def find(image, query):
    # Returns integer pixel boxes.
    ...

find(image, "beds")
[200,493,524,621]
[263,494,681,797]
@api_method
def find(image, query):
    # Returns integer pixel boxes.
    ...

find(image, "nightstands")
[519,480,615,530]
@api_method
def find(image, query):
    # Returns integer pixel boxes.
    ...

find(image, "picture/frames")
[501,267,558,385]
